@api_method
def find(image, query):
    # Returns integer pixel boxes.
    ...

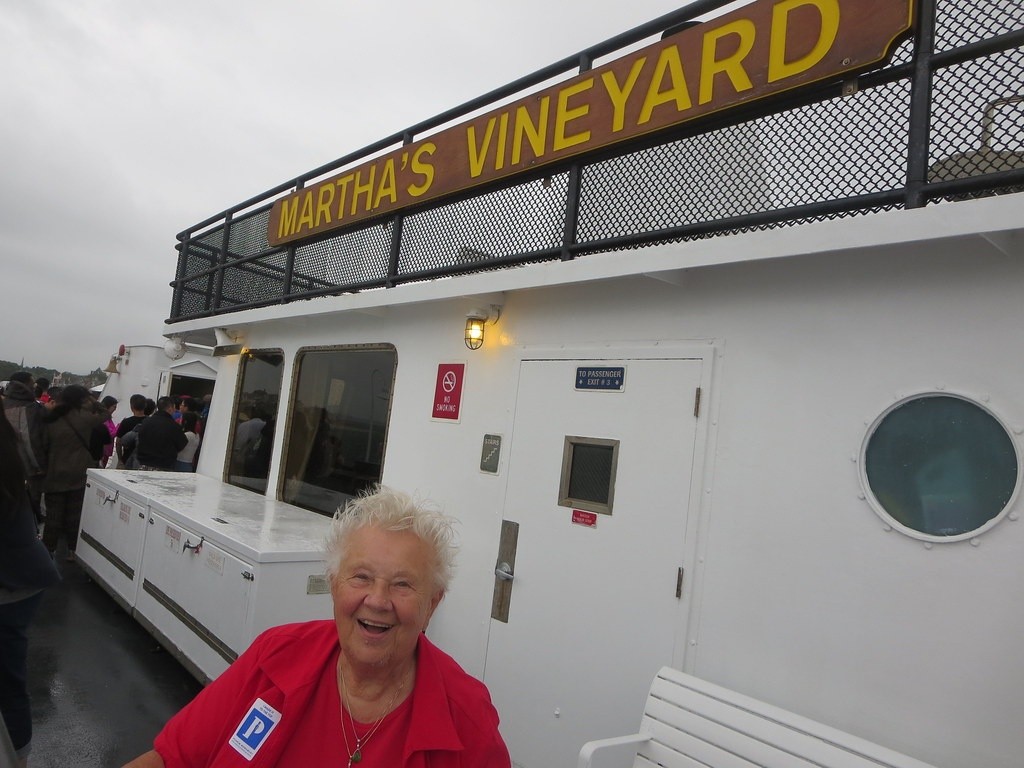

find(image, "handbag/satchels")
[26,536,62,589]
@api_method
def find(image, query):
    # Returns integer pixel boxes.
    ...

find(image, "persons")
[0,372,121,758]
[229,389,384,497]
[116,394,212,472]
[119,482,511,768]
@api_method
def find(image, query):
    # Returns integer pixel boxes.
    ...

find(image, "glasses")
[25,382,38,389]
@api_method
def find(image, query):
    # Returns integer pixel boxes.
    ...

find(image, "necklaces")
[339,657,410,768]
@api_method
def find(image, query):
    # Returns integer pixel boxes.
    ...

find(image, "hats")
[60,385,87,401]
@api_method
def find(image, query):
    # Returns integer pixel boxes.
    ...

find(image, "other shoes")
[67,550,75,562]
